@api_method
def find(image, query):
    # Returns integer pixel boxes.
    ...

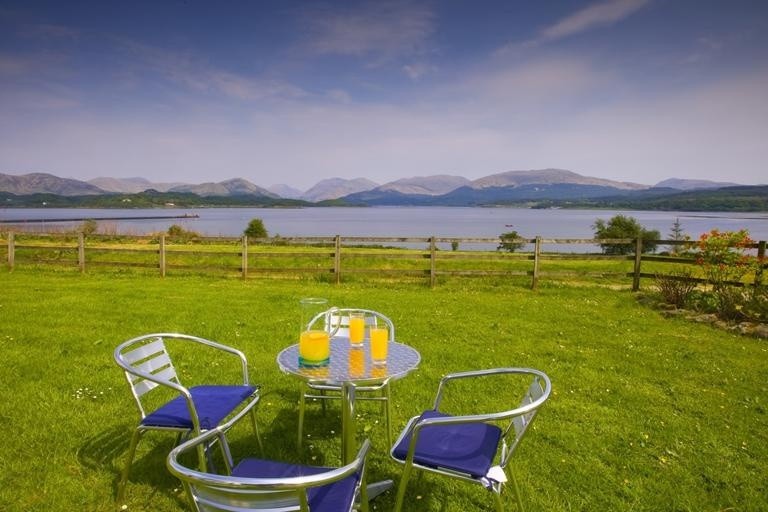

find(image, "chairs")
[389,366,552,509]
[162,429,374,512]
[294,308,397,457]
[112,331,262,503]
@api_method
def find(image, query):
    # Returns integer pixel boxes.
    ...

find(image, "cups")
[347,310,365,348]
[348,347,365,379]
[368,323,388,365]
[298,296,343,368]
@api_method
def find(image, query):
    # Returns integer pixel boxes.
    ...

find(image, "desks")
[276,336,422,506]
